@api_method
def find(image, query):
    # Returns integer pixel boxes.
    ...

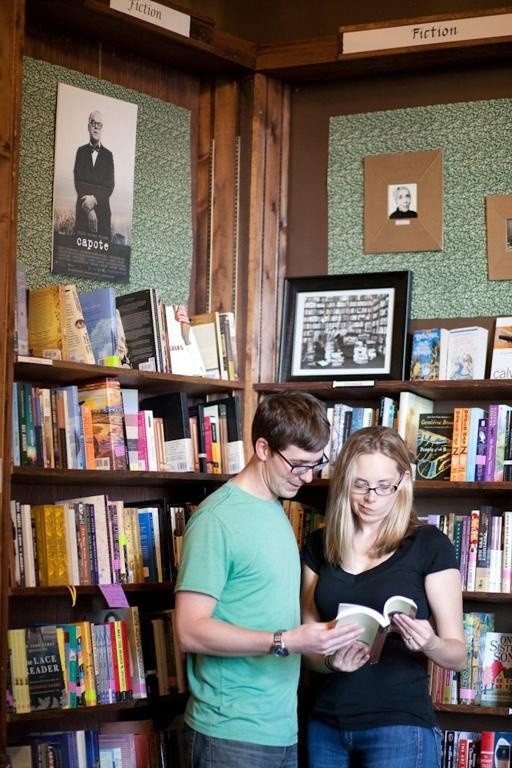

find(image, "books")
[283,500,512,592]
[410,317,511,379]
[335,594,418,665]
[8,715,183,767]
[8,607,188,716]
[318,391,511,483]
[10,494,207,588]
[426,612,512,708]
[12,378,246,475]
[440,731,512,768]
[7,263,239,382]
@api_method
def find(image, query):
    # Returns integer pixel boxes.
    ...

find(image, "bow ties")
[89,145,100,153]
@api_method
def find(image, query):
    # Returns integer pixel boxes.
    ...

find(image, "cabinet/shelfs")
[14,346,512,765]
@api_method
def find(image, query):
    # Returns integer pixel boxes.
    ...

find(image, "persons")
[387,185,418,219]
[69,107,116,240]
[172,390,366,768]
[294,425,465,767]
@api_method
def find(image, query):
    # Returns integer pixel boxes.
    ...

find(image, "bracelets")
[324,657,337,672]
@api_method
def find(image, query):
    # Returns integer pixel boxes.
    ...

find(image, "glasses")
[89,120,102,129]
[350,473,404,496]
[275,448,329,475]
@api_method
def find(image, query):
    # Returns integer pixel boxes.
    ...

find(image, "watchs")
[272,629,289,658]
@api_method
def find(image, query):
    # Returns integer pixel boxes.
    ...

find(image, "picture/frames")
[362,148,512,282]
[282,271,411,379]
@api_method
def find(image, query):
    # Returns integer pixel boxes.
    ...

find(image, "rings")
[406,637,413,640]
